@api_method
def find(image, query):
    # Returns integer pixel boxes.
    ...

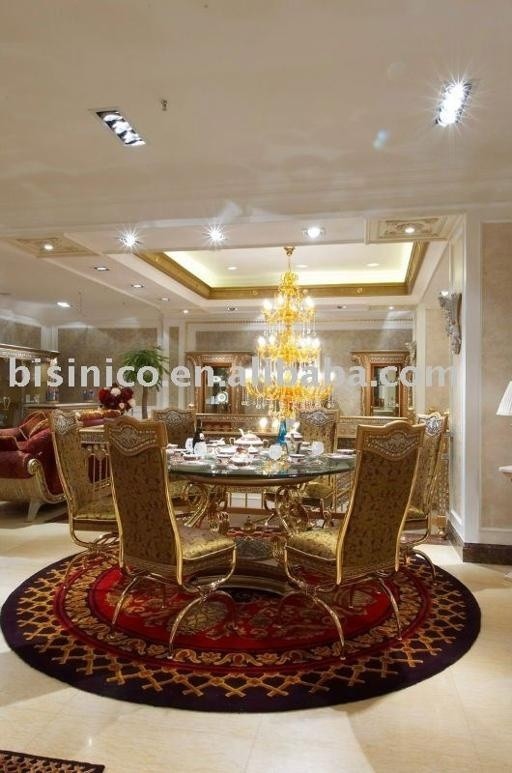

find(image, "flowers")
[98,384,133,412]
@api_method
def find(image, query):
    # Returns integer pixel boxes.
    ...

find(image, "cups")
[217,455,228,464]
[183,455,201,461]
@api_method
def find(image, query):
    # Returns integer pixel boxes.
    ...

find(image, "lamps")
[241,247,332,422]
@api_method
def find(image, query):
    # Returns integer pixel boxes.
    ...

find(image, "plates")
[170,460,210,471]
[215,463,228,469]
[325,453,354,459]
[338,448,355,454]
[290,454,304,459]
[171,448,186,452]
[217,446,234,453]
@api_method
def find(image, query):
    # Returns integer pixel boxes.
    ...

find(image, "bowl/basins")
[234,428,263,452]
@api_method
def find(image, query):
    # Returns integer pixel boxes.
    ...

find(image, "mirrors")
[185,351,253,414]
[351,350,410,417]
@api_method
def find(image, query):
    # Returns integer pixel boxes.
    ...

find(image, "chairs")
[153,409,228,528]
[103,416,238,659]
[50,410,197,608]
[274,420,425,661]
[263,407,337,533]
[400,411,448,586]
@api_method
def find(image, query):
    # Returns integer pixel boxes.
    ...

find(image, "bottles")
[193,419,204,453]
[278,416,288,445]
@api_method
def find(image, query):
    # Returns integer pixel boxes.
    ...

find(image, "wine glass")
[310,442,324,466]
[185,437,194,453]
[270,446,282,467]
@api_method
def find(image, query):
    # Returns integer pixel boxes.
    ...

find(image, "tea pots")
[284,426,303,454]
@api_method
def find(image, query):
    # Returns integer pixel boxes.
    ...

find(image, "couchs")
[1,409,122,522]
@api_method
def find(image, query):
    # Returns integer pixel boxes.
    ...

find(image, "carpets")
[0,750,105,773]
[0,526,481,713]
